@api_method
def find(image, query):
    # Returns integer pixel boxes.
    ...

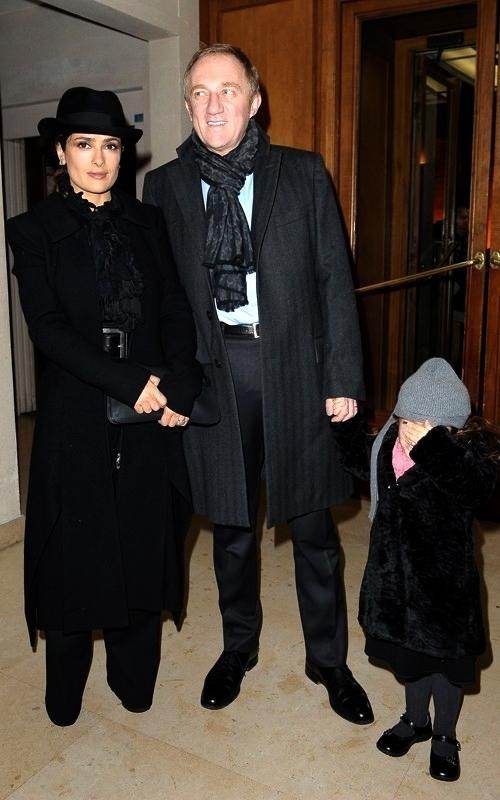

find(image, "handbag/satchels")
[106,368,221,426]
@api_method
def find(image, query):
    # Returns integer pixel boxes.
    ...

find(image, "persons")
[5,86,200,728]
[356,357,500,783]
[142,44,374,726]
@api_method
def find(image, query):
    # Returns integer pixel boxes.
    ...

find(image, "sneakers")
[429,735,461,781]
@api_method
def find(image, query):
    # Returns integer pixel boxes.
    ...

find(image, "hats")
[393,357,471,430]
[38,87,143,142]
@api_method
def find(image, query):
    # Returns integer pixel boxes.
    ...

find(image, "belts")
[220,323,260,340]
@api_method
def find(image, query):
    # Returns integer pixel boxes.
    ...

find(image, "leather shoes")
[201,650,261,710]
[305,658,374,725]
[376,713,432,757]
